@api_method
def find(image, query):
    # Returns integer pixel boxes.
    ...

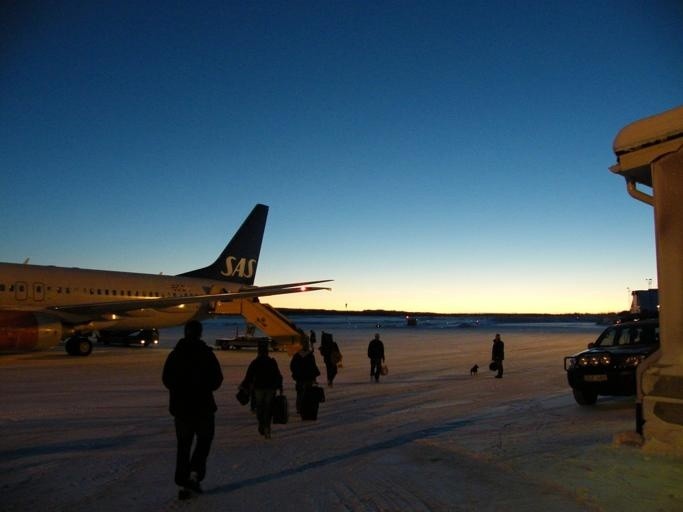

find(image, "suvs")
[563,310,660,406]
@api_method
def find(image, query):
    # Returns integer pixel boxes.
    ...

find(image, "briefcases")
[272,391,290,426]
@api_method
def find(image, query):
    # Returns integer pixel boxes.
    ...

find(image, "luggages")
[301,382,325,420]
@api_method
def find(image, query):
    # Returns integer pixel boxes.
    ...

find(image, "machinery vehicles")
[99,327,159,345]
[212,299,307,351]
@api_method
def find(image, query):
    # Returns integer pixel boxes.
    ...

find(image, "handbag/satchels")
[380,364,387,376]
[303,386,324,402]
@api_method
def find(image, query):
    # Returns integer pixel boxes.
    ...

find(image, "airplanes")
[0,203,334,356]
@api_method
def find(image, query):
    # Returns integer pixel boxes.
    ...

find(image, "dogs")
[470,364,479,376]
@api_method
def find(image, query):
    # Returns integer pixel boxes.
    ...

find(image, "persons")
[320,331,326,345]
[491,333,504,378]
[288,321,296,330]
[296,327,304,335]
[367,333,385,383]
[319,331,343,387]
[221,287,227,293]
[288,336,320,421]
[236,337,283,439]
[309,329,316,352]
[160,318,224,501]
